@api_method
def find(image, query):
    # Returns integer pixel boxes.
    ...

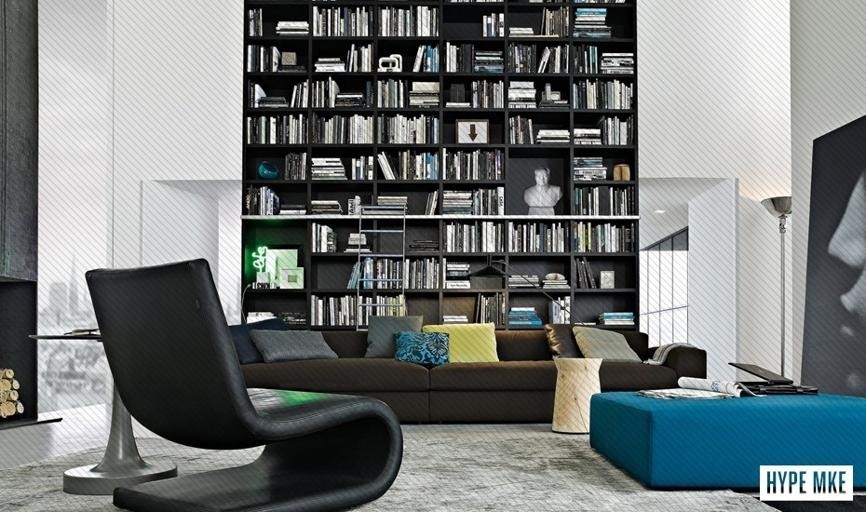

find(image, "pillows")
[422,323,498,363]
[229,319,288,364]
[250,330,338,363]
[365,314,423,357]
[394,331,449,366]
[572,326,643,363]
[544,324,581,357]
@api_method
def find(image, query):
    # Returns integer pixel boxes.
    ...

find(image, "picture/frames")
[455,118,488,143]
[280,267,303,290]
[800,114,866,398]
[264,244,305,287]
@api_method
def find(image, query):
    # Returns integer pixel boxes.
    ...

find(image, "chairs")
[87,257,401,512]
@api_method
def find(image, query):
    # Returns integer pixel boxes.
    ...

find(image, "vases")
[553,355,605,434]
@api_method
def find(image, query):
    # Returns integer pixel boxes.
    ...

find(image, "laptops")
[729,363,794,384]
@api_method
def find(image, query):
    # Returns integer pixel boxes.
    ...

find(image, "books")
[443,187,504,215]
[378,114,439,144]
[412,44,439,73]
[398,150,439,180]
[573,8,611,38]
[509,222,570,252]
[543,278,570,289]
[508,274,539,288]
[529,0,625,3]
[576,257,597,288]
[312,200,341,214]
[365,81,374,108]
[508,81,537,109]
[314,56,346,72]
[536,129,570,143]
[312,113,374,144]
[311,295,407,325]
[312,157,348,180]
[284,152,306,180]
[510,305,542,325]
[378,195,408,215]
[472,81,504,108]
[352,156,374,180]
[249,8,263,37]
[538,44,569,73]
[601,52,635,74]
[451,0,504,2]
[409,239,439,252]
[507,42,536,74]
[410,82,440,108]
[248,45,281,72]
[574,222,635,252]
[335,91,364,107]
[576,185,635,216]
[312,223,337,252]
[246,186,307,215]
[343,233,371,253]
[260,96,288,108]
[730,362,818,394]
[602,115,634,146]
[377,79,408,108]
[345,44,371,72]
[443,148,504,180]
[311,76,340,108]
[548,296,571,324]
[573,79,633,109]
[510,115,534,144]
[573,157,608,181]
[599,313,635,325]
[575,322,597,326]
[445,102,471,107]
[574,128,602,145]
[363,257,440,289]
[639,377,767,399]
[474,50,504,73]
[475,292,505,325]
[573,45,598,74]
[424,190,438,215]
[443,315,468,324]
[508,26,533,37]
[443,258,471,289]
[446,42,474,72]
[378,151,396,180]
[313,6,439,37]
[289,79,308,108]
[276,21,309,35]
[447,222,504,252]
[247,114,308,144]
[249,83,266,108]
[539,83,570,109]
[541,7,568,37]
[483,13,504,38]
[348,261,363,289]
[247,312,306,325]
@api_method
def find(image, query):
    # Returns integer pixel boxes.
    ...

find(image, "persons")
[827,165,865,394]
[525,169,562,206]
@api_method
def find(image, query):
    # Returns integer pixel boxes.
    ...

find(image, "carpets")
[2,421,781,511]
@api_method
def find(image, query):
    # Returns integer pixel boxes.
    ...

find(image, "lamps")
[760,195,792,376]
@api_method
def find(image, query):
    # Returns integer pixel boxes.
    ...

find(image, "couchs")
[431,323,708,422]
[227,317,430,423]
[590,389,865,492]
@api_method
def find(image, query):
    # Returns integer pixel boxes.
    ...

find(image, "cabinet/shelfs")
[243,0,637,327]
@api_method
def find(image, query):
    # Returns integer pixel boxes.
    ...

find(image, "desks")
[29,334,179,494]
